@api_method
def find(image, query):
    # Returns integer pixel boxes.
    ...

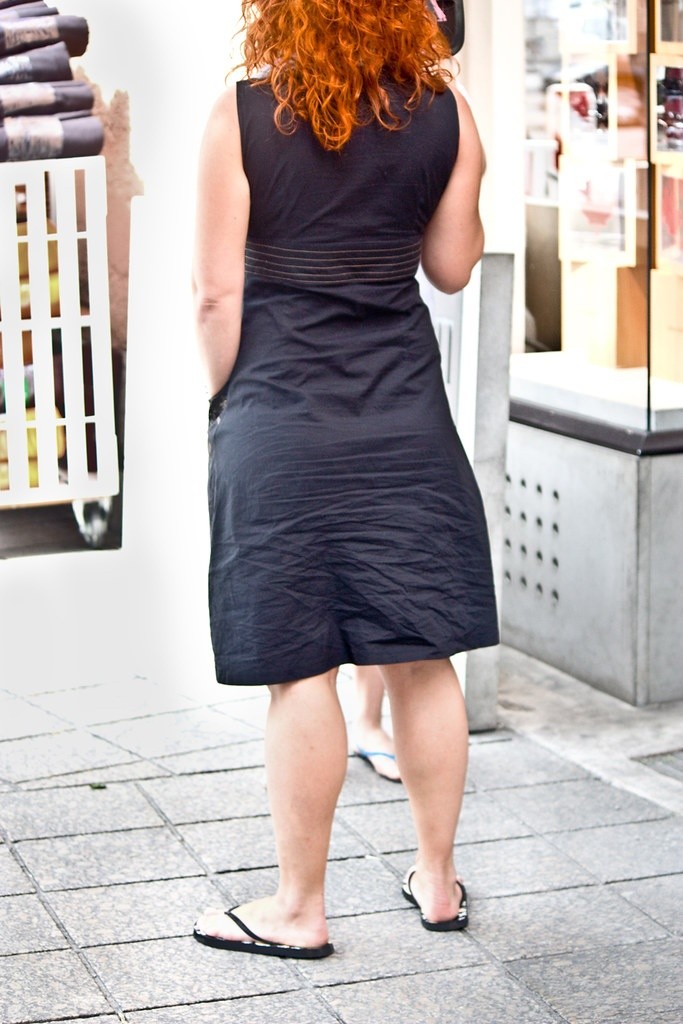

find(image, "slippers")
[351,728,403,782]
[401,871,468,932]
[194,905,335,959]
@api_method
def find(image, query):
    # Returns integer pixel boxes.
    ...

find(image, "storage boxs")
[0,154,119,508]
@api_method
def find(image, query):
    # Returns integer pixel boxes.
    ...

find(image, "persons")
[195,0,486,959]
